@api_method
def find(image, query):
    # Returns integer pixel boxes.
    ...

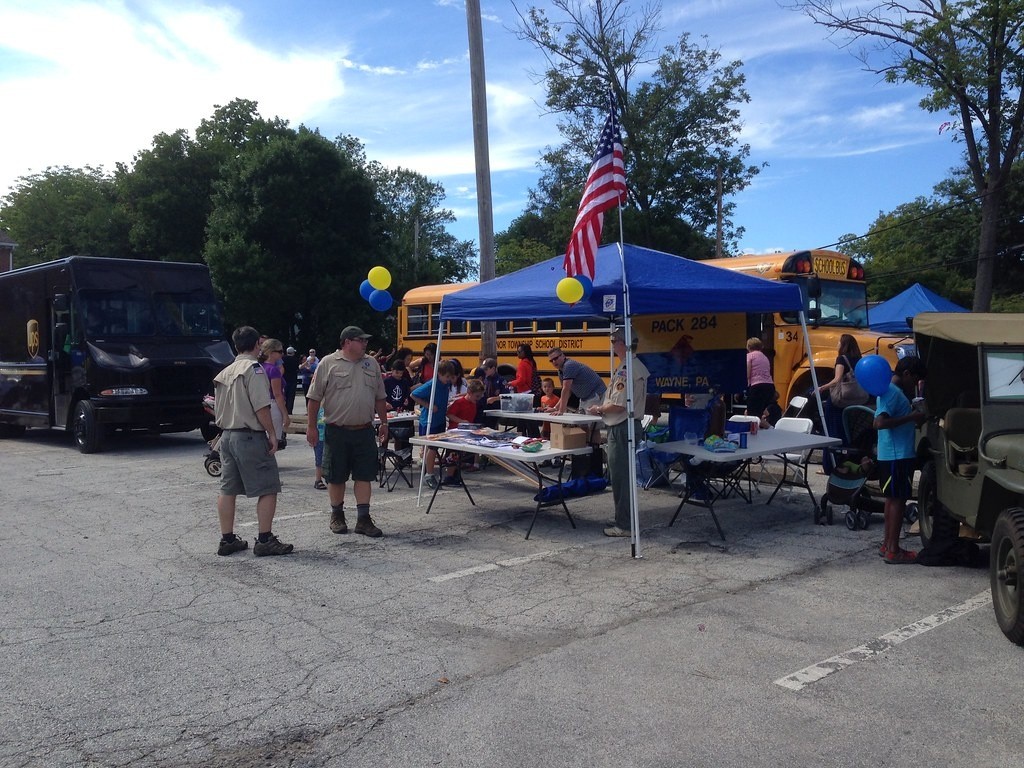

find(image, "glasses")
[549,353,562,363]
[352,338,368,343]
[611,340,620,344]
[272,349,283,354]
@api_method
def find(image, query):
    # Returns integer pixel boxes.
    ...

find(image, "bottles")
[316,418,326,442]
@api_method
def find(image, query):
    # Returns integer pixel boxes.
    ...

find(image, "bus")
[0,255,238,454]
[397,250,915,424]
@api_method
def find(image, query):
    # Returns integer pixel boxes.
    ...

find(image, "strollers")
[813,405,920,530]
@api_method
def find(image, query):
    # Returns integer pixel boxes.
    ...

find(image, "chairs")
[751,418,814,503]
[727,415,761,499]
[706,419,752,504]
[781,395,808,418]
[643,407,712,498]
[945,407,982,480]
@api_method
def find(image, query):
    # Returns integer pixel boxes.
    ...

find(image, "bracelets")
[379,422,388,427]
[553,406,557,410]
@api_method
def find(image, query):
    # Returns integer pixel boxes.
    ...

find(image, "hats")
[610,326,639,344]
[287,347,296,352]
[466,367,485,379]
[480,358,496,369]
[340,326,373,345]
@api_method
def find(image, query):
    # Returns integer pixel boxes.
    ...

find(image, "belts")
[326,421,372,430]
[225,428,266,434]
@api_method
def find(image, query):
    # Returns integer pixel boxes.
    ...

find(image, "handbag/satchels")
[412,375,425,385]
[830,380,869,408]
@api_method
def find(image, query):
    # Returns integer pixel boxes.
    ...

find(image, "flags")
[563,91,629,308]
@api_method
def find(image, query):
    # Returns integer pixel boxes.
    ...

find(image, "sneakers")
[355,518,382,537]
[603,526,641,537]
[426,475,438,489]
[217,538,248,555]
[877,543,918,564]
[254,536,293,555]
[330,512,347,533]
[606,518,616,527]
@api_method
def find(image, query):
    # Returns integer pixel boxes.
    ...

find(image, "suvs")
[902,311,1023,642]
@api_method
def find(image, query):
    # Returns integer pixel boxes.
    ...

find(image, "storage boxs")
[500,393,535,413]
[550,423,586,450]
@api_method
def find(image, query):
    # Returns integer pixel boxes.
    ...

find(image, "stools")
[378,445,414,492]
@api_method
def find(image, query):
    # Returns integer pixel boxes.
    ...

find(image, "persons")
[585,325,651,537]
[277,339,508,473]
[258,338,290,454]
[447,379,485,484]
[211,327,295,558]
[543,348,607,446]
[812,334,866,475]
[506,343,541,438]
[538,377,563,468]
[305,326,389,537]
[407,360,455,488]
[874,354,931,564]
[314,398,327,490]
[746,337,774,431]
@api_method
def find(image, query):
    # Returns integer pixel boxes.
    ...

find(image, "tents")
[861,282,974,334]
[415,241,847,556]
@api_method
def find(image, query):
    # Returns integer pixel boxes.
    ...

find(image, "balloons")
[359,279,377,300]
[556,277,584,305]
[854,354,892,398]
[571,274,592,302]
[368,266,392,289]
[369,289,392,312]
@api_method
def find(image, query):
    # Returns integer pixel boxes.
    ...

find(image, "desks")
[409,427,592,539]
[373,410,421,425]
[486,410,602,446]
[653,428,843,541]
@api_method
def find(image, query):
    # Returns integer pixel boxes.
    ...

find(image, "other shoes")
[446,475,460,487]
[816,468,825,474]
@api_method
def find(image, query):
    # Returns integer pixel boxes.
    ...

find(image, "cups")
[739,432,747,448]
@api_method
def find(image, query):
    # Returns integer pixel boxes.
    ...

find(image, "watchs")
[596,405,601,416]
